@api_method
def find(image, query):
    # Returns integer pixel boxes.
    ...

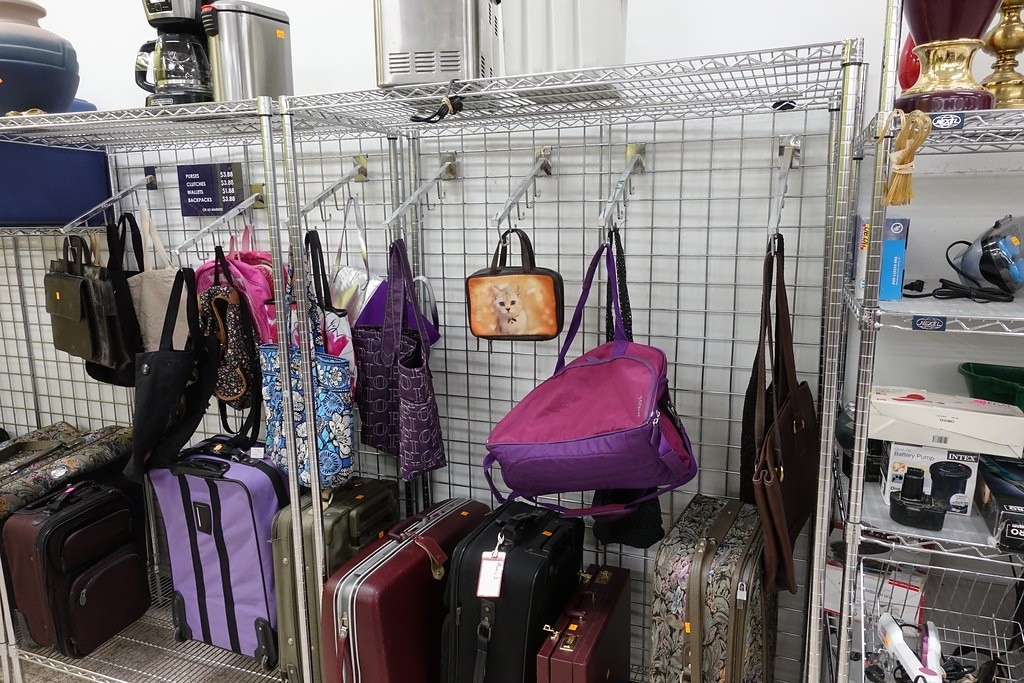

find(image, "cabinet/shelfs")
[0,35,872,683]
[815,0,1024,683]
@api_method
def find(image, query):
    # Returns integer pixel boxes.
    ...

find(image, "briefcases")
[535,563,631,683]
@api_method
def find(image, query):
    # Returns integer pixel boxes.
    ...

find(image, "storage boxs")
[866,381,1024,461]
[977,454,1024,555]
[823,524,932,634]
[880,441,980,517]
[854,216,910,299]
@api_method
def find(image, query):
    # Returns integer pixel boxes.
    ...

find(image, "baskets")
[859,557,1024,683]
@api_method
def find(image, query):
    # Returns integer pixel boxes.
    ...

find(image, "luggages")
[270,475,400,683]
[2,481,151,659]
[648,494,778,683]
[140,435,290,670]
[432,501,585,683]
[322,496,492,683]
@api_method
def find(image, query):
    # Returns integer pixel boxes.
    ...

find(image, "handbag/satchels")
[740,235,820,594]
[44,202,446,488]
[465,230,564,340]
[464,227,696,550]
[0,420,133,610]
[590,489,665,549]
